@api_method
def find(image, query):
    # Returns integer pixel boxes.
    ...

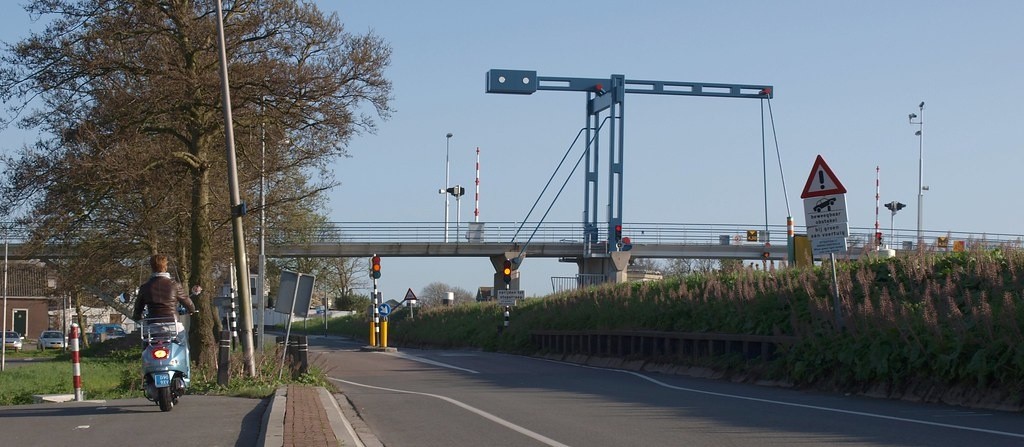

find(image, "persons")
[133,255,195,385]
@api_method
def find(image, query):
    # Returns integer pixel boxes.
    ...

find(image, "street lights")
[257,95,290,357]
[445,132,453,244]
[909,101,925,246]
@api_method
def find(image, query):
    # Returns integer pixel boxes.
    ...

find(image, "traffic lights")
[615,224,621,242]
[503,260,512,283]
[372,256,381,279]
[885,202,894,210]
[895,201,906,210]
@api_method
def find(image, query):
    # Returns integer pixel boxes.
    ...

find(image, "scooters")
[119,284,202,410]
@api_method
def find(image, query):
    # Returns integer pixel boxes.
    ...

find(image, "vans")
[93,323,126,343]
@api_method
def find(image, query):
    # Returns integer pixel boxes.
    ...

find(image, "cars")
[37,330,93,351]
[0,331,25,352]
